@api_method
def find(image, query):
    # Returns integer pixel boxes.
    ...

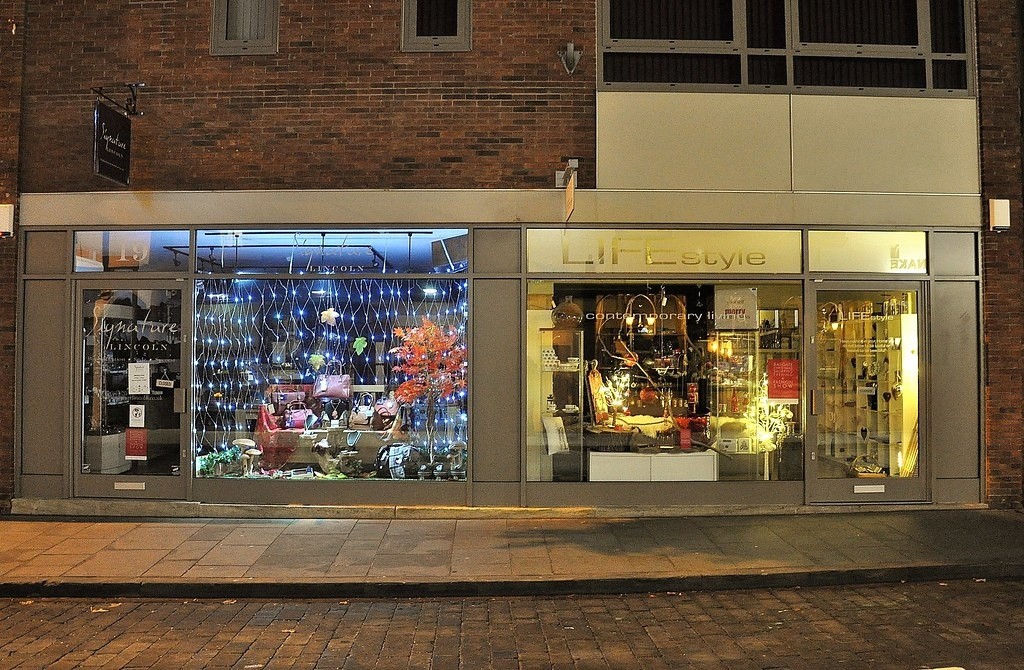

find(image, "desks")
[585,448,720,481]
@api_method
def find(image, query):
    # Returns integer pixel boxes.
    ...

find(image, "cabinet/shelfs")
[594,294,687,369]
[538,328,584,482]
[816,315,919,477]
[85,319,179,402]
[709,348,800,392]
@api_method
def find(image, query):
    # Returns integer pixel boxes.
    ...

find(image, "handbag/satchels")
[350,392,375,425]
[283,402,314,427]
[373,408,394,430]
[375,398,398,416]
[312,359,353,399]
[271,392,305,415]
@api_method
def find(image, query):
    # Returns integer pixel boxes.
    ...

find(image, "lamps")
[173,252,181,266]
[372,254,379,268]
[209,248,217,265]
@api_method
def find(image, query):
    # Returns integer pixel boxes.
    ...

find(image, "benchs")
[235,385,457,469]
